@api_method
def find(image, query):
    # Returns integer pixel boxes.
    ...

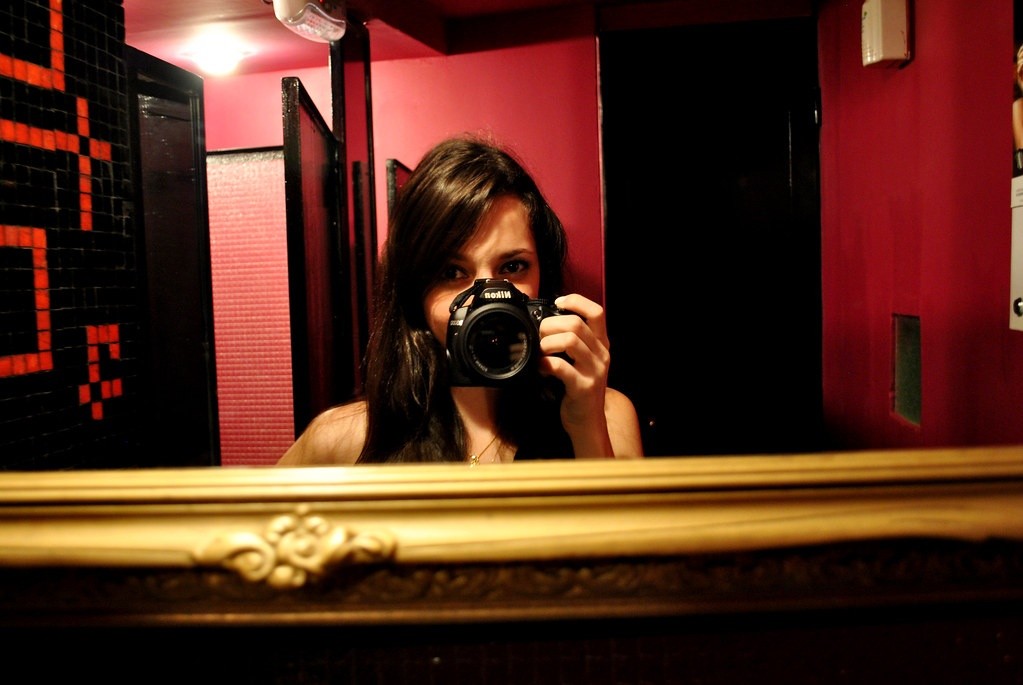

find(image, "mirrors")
[0,0,1023,635]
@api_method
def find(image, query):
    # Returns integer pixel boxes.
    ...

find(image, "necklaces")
[468,434,498,467]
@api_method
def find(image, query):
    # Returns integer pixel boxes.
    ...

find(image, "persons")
[274,137,642,470]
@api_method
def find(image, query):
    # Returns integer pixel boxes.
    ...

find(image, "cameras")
[444,279,586,388]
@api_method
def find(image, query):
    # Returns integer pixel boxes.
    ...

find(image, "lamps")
[271,0,348,45]
